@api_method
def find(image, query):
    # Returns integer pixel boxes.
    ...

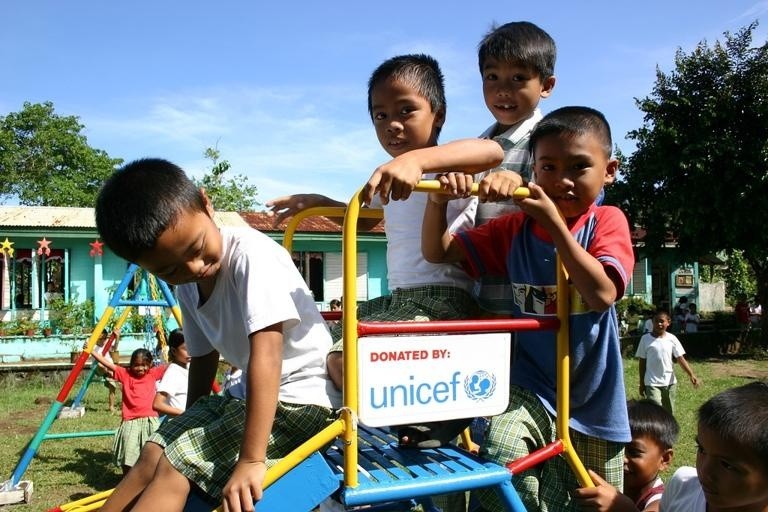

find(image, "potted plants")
[0,294,165,336]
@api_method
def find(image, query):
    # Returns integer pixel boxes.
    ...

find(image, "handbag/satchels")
[674,306,683,314]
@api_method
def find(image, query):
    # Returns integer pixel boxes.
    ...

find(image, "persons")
[90,327,122,416]
[266,51,508,450]
[569,397,680,511]
[634,308,700,418]
[43,281,64,309]
[96,157,348,511]
[734,293,752,347]
[655,379,768,512]
[619,294,702,357]
[749,295,762,315]
[83,340,174,480]
[155,329,170,359]
[472,19,560,324]
[418,106,639,511]
[151,328,192,428]
[330,300,340,311]
